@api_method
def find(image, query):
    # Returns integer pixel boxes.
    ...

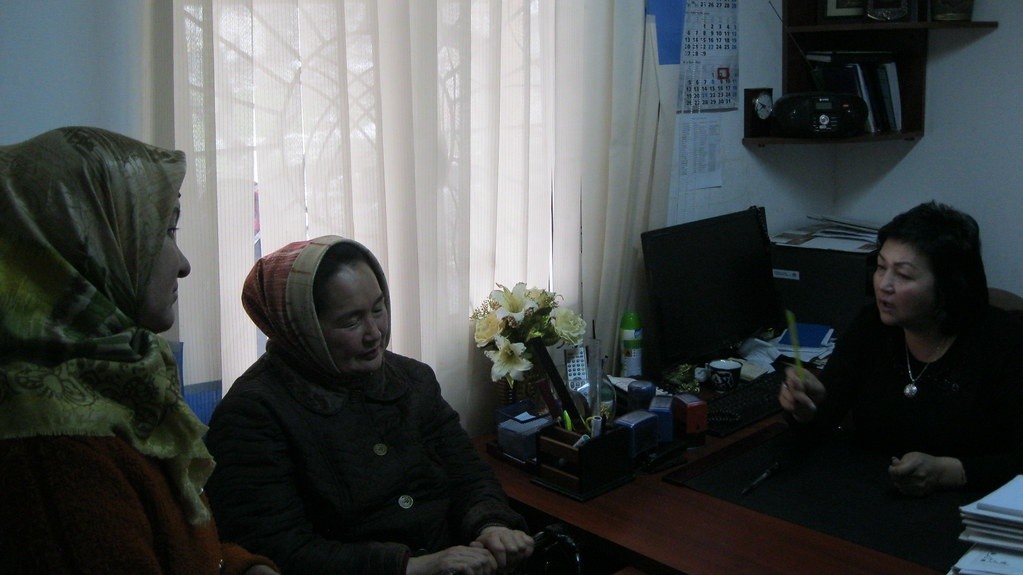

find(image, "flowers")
[469,283,586,390]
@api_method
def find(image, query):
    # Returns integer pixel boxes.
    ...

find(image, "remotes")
[564,347,590,409]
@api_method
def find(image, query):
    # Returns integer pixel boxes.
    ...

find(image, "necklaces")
[904,339,945,397]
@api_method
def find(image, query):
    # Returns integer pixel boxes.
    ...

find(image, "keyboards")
[704,372,813,440]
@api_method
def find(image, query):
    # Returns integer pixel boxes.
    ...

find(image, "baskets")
[666,346,795,439]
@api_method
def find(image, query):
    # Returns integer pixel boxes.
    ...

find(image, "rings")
[783,383,788,388]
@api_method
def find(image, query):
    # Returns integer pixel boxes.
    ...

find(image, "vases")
[488,364,538,439]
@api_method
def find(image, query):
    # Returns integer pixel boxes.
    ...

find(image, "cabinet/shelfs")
[743,0,997,148]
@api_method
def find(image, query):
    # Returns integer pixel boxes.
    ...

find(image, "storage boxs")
[772,223,879,344]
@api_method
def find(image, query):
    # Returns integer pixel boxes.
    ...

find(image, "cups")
[708,359,743,394]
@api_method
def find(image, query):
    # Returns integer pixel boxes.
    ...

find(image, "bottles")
[617,311,642,378]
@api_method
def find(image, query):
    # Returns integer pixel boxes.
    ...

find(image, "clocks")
[755,93,773,120]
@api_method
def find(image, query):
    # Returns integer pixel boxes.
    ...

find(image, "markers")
[572,434,590,451]
[591,416,602,440]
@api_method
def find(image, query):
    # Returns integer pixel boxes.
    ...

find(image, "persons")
[0,127,277,575]
[779,201,1023,568]
[206,235,535,575]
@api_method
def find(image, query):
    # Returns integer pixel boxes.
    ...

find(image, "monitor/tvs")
[641,205,786,370]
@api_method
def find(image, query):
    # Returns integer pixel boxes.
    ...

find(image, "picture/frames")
[816,0,865,24]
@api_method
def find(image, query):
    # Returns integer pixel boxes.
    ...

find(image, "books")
[847,61,901,132]
[947,475,1023,575]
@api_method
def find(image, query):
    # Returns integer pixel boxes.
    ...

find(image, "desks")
[467,382,947,575]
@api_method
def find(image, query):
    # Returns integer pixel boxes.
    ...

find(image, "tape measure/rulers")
[588,338,602,428]
